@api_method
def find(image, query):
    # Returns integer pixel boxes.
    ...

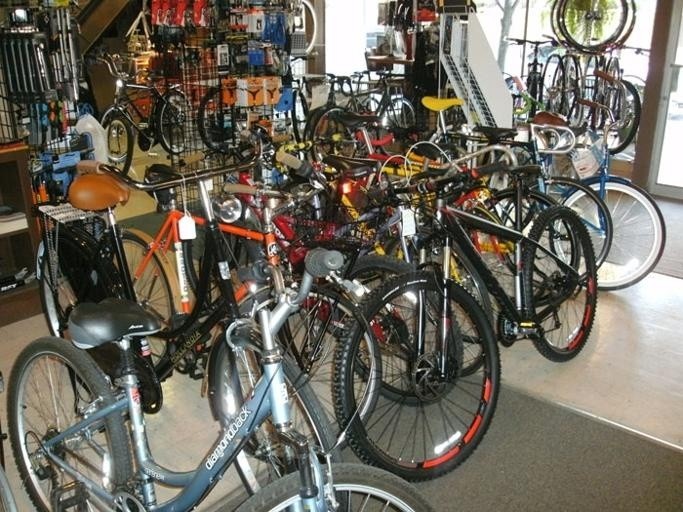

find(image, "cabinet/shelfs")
[0,142,58,330]
